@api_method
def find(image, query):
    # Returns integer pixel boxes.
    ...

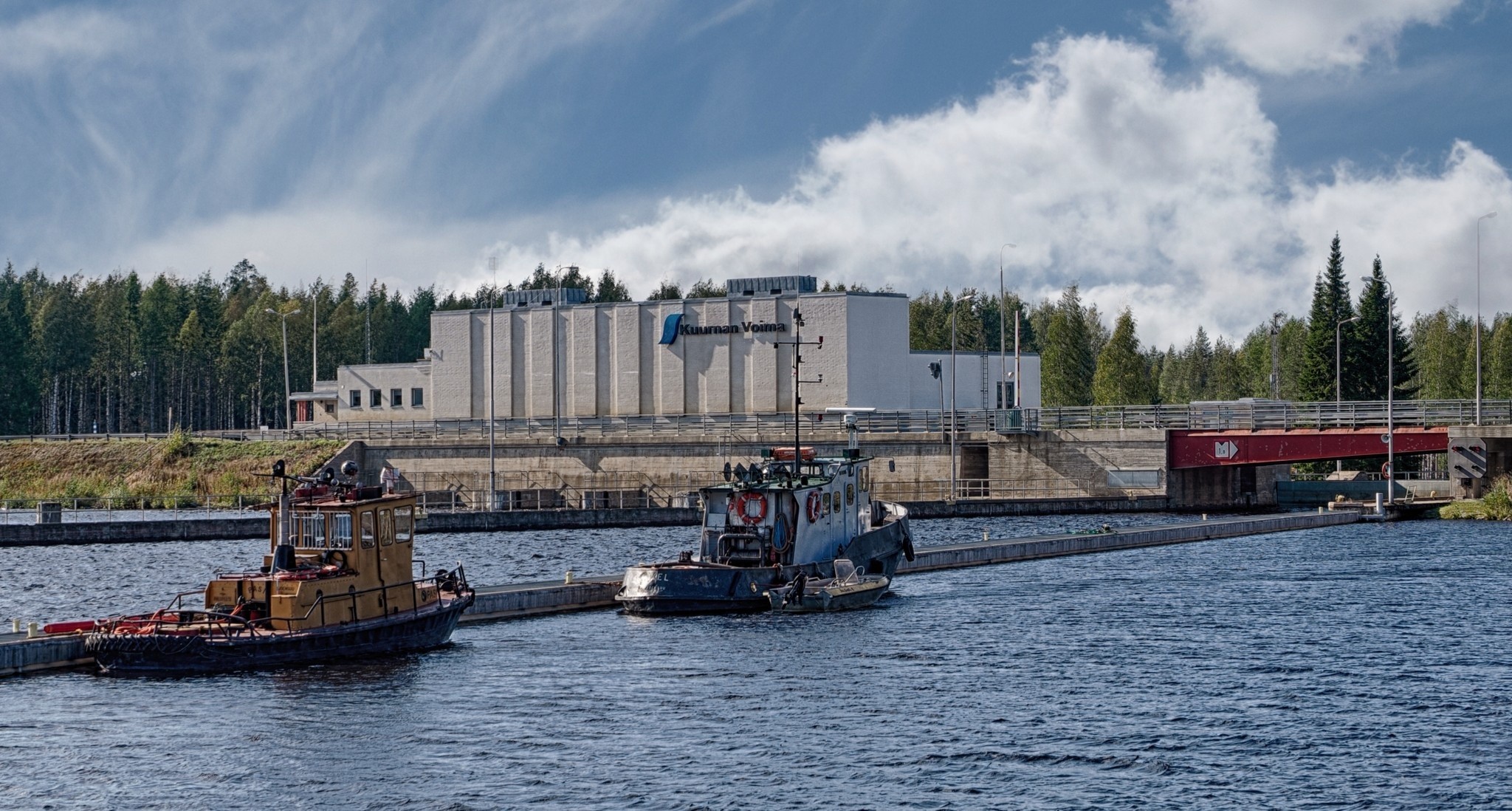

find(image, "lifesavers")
[807,491,820,523]
[275,564,337,580]
[1382,462,1389,479]
[736,493,767,523]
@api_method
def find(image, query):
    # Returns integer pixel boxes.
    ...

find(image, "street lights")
[312,294,317,386]
[1358,274,1395,505]
[999,242,1018,410]
[1336,316,1362,472]
[1475,210,1497,426]
[949,294,974,501]
[265,308,301,441]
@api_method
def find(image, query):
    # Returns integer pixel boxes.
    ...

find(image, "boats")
[67,436,479,681]
[613,301,914,619]
[768,557,892,617]
